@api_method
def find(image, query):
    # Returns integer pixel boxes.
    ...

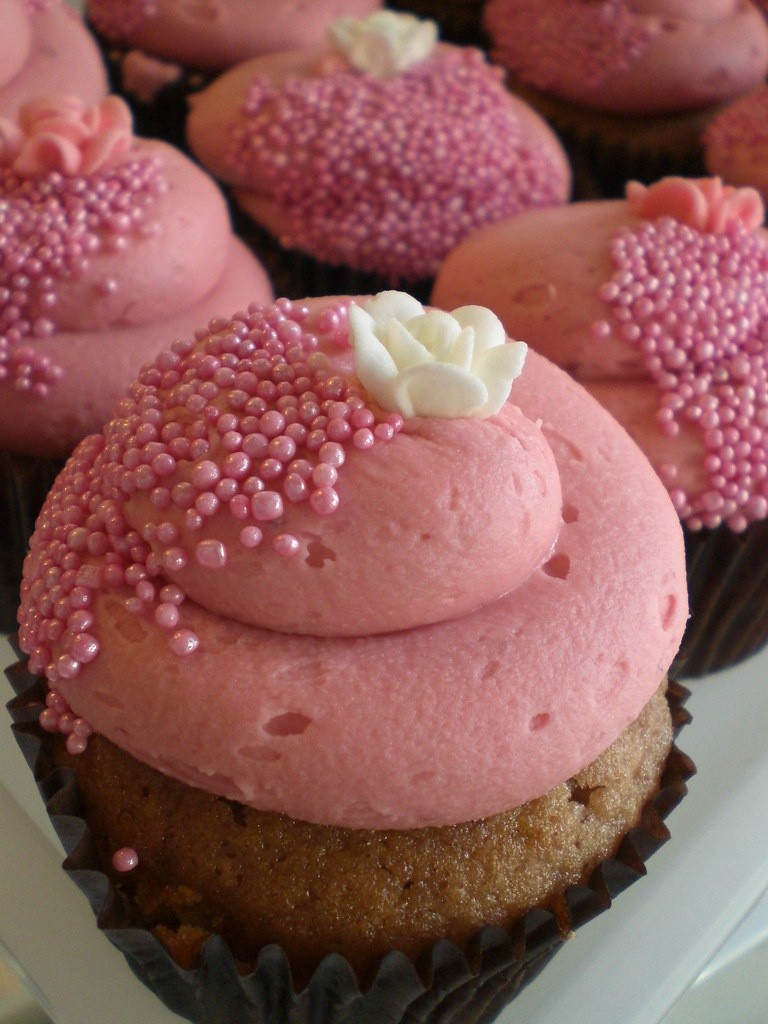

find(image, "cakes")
[0,0,766,1023]
